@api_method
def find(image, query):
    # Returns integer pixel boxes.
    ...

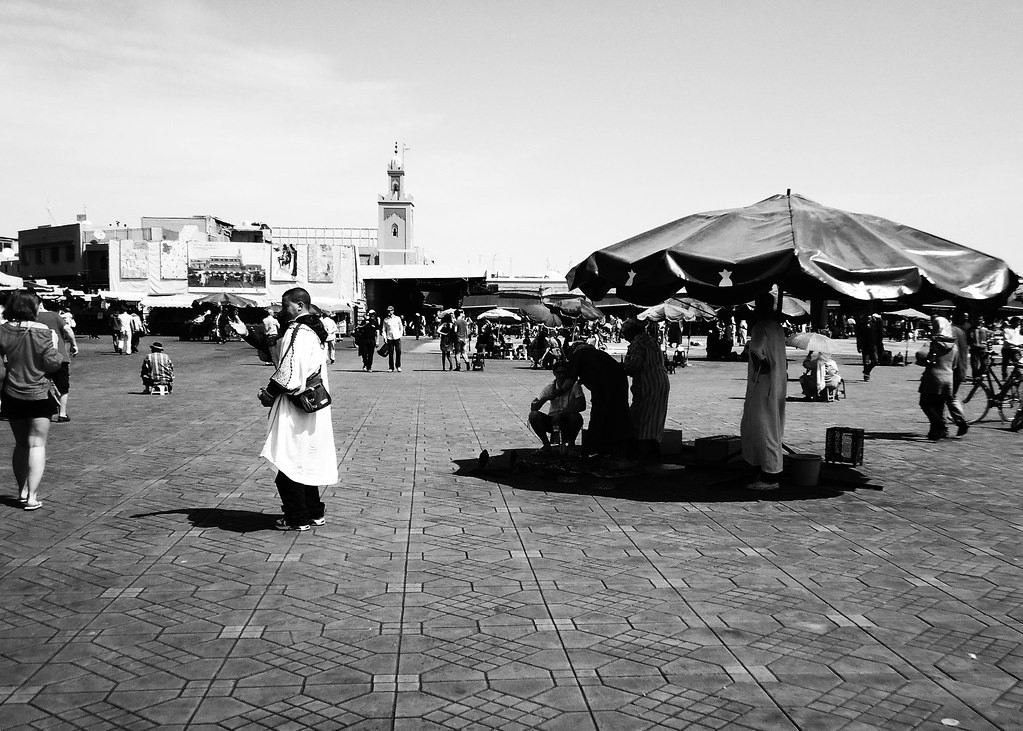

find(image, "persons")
[262,309,338,365]
[228,287,339,529]
[184,304,242,343]
[438,309,505,372]
[724,314,856,342]
[856,312,1023,440]
[110,306,146,354]
[736,291,790,493]
[801,349,842,402]
[0,290,78,511]
[398,310,442,340]
[352,305,405,370]
[524,319,671,468]
[142,341,174,394]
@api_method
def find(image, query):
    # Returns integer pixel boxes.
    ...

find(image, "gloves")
[258,387,275,407]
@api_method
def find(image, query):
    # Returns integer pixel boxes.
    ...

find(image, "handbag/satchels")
[378,345,389,357]
[350,327,364,339]
[915,340,937,366]
[286,373,331,413]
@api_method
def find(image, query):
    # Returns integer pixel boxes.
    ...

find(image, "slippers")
[25,500,43,510]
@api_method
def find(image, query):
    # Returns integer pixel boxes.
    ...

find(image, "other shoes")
[957,421,968,437]
[466,362,470,370]
[397,367,402,372]
[331,359,334,365]
[143,390,150,394]
[389,368,393,372]
[454,367,460,371]
[131,346,138,353]
[364,366,368,370]
[58,413,71,423]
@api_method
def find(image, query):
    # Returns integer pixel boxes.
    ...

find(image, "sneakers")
[275,517,311,531]
[310,517,325,527]
[749,482,780,490]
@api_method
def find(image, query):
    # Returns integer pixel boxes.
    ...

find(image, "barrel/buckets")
[787,453,823,487]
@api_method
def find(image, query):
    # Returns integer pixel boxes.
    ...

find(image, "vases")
[788,453,821,485]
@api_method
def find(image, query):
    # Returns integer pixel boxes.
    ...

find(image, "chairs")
[663,349,686,374]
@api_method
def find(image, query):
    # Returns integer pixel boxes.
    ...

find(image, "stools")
[151,385,169,396]
[471,346,528,370]
[824,387,837,402]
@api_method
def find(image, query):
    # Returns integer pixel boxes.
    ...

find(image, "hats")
[368,310,377,314]
[387,306,394,311]
[150,342,164,352]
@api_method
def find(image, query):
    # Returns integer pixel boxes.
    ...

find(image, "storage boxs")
[695,435,741,463]
[826,426,863,464]
[663,429,683,452]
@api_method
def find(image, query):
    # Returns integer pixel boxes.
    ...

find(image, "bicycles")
[946,351,1023,424]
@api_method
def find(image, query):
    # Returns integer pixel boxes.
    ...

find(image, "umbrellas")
[635,297,717,365]
[563,186,1021,322]
[517,304,562,330]
[192,292,260,311]
[553,297,606,342]
[786,331,842,355]
[477,309,521,340]
[885,307,931,323]
